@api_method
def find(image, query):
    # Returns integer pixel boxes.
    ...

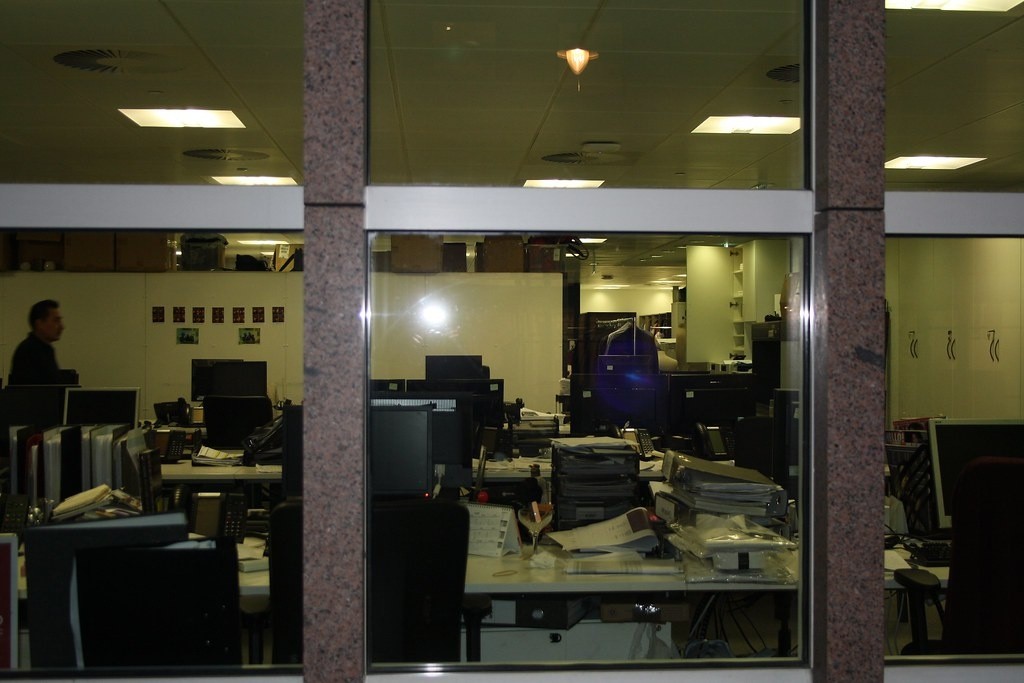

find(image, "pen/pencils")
[27,497,55,526]
[477,444,488,478]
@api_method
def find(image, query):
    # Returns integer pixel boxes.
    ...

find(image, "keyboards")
[904,540,952,567]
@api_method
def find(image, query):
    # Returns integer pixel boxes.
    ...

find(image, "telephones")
[148,429,187,461]
[0,492,30,551]
[611,423,657,461]
[695,422,738,461]
[173,483,249,545]
[473,420,514,459]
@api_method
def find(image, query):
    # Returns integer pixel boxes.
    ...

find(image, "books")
[140,448,164,516]
[52,484,141,523]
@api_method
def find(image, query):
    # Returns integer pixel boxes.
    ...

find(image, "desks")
[148,421,951,666]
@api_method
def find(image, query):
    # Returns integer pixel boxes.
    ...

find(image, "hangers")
[596,316,638,329]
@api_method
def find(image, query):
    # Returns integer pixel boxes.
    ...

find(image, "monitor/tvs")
[927,417,1024,529]
[283,355,504,501]
[570,354,802,500]
[0,385,141,431]
[190,358,267,401]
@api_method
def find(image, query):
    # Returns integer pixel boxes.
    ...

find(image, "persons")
[8,300,65,384]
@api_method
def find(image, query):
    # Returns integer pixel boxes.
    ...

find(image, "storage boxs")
[369,229,525,273]
[1,226,227,273]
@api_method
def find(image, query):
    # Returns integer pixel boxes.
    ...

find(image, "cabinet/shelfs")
[670,239,792,376]
